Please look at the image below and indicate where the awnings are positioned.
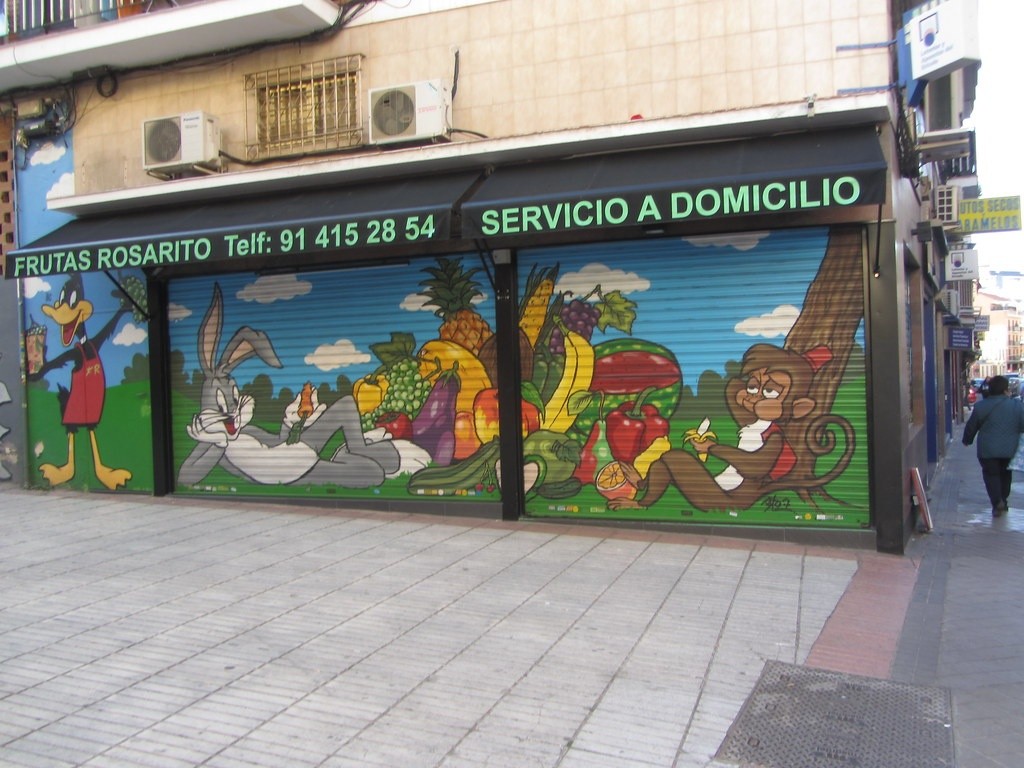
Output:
[4,167,486,281]
[455,122,887,250]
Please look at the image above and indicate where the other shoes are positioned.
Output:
[992,502,1008,517]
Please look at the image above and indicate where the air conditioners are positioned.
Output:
[367,80,454,148]
[934,184,965,226]
[141,109,224,176]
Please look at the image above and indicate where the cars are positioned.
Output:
[967,373,1024,405]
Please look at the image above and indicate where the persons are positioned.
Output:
[962,375,1024,517]
[978,375,991,400]
